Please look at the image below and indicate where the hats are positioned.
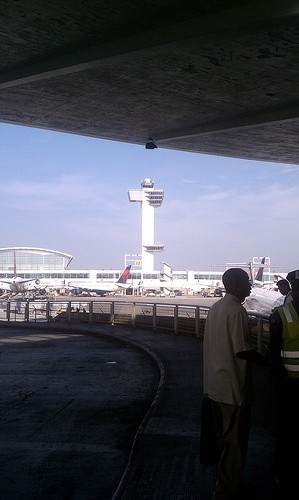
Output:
[286,270,299,285]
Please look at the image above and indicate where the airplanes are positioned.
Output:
[0,275,40,301]
[41,264,135,299]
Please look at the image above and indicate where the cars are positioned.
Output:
[145,291,175,299]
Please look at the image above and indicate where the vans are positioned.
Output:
[213,289,223,298]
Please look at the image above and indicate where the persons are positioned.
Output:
[202,269,270,500]
[268,270,299,500]
[276,279,292,304]
